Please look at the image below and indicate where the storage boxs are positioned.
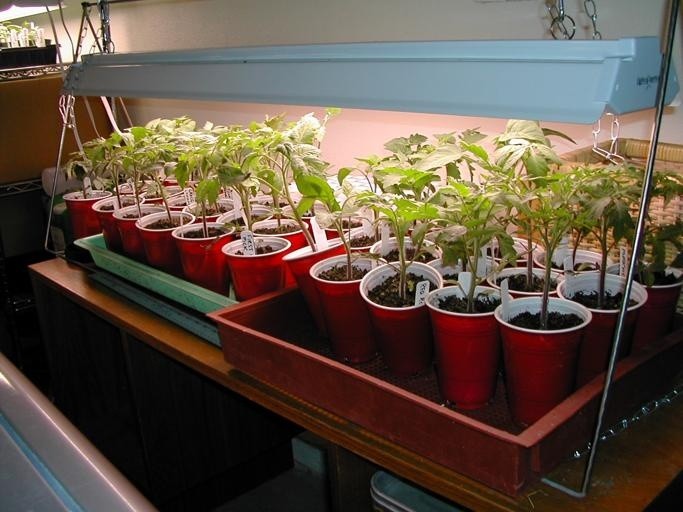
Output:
[0,40,61,69]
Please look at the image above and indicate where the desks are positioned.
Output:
[27,257,681,511]
